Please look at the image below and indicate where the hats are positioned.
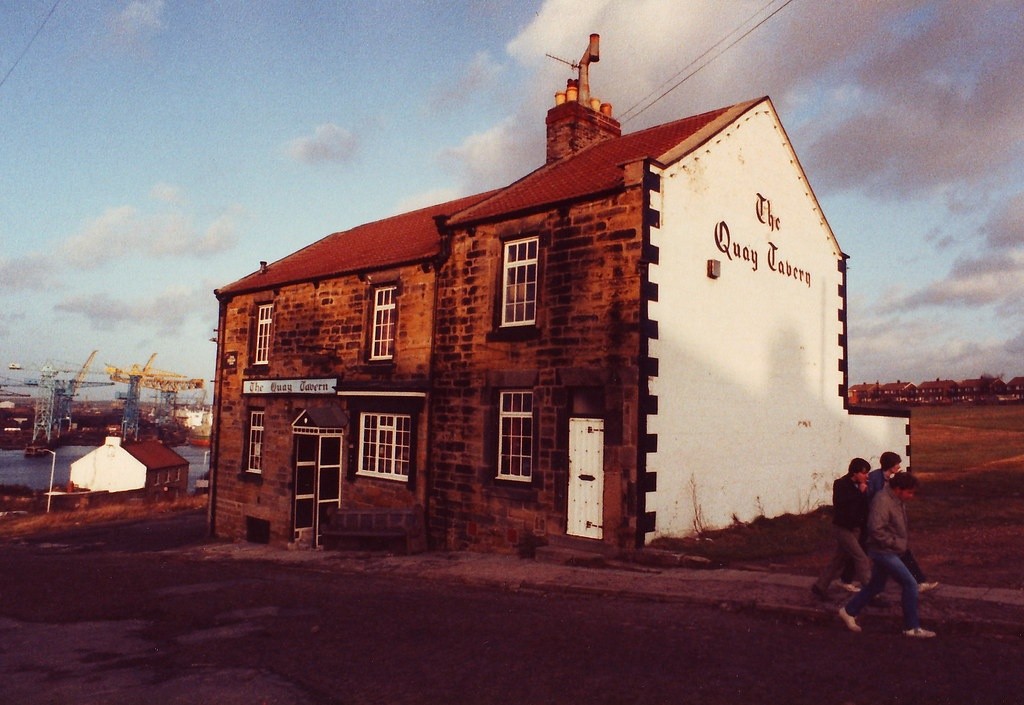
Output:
[881,452,902,470]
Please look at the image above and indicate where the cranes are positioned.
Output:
[1,350,205,459]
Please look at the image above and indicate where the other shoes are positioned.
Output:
[812,584,832,602]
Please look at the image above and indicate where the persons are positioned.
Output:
[839,473,936,637]
[839,452,939,593]
[811,458,892,606]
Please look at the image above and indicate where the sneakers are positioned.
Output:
[902,627,936,639]
[918,581,938,593]
[836,579,861,592]
[837,608,862,632]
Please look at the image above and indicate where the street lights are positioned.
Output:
[41,448,56,515]
